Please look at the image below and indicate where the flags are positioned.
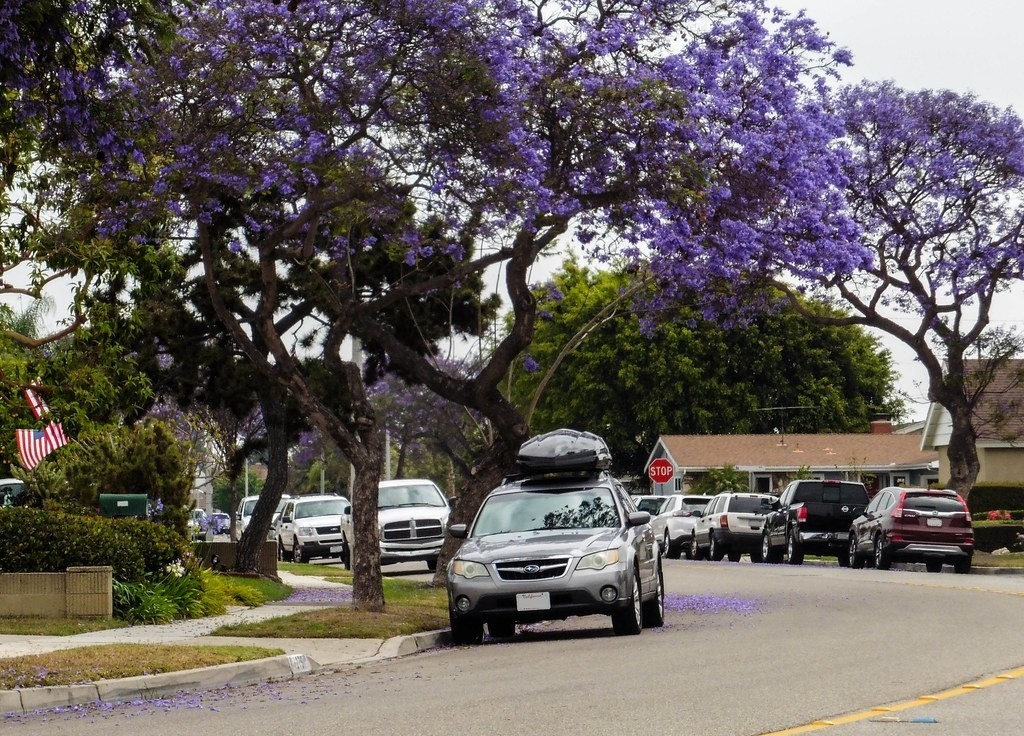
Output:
[15,430,69,473]
[25,379,71,450]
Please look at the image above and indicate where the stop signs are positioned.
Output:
[648,457,673,485]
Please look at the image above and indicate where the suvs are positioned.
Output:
[761,479,872,569]
[235,493,292,544]
[690,490,779,563]
[446,429,665,644]
[338,478,452,572]
[846,486,976,575]
[270,493,352,564]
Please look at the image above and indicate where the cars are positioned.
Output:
[630,493,669,521]
[650,494,714,559]
[212,512,232,533]
[188,509,210,537]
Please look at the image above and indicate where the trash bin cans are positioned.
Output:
[99,493,148,520]
